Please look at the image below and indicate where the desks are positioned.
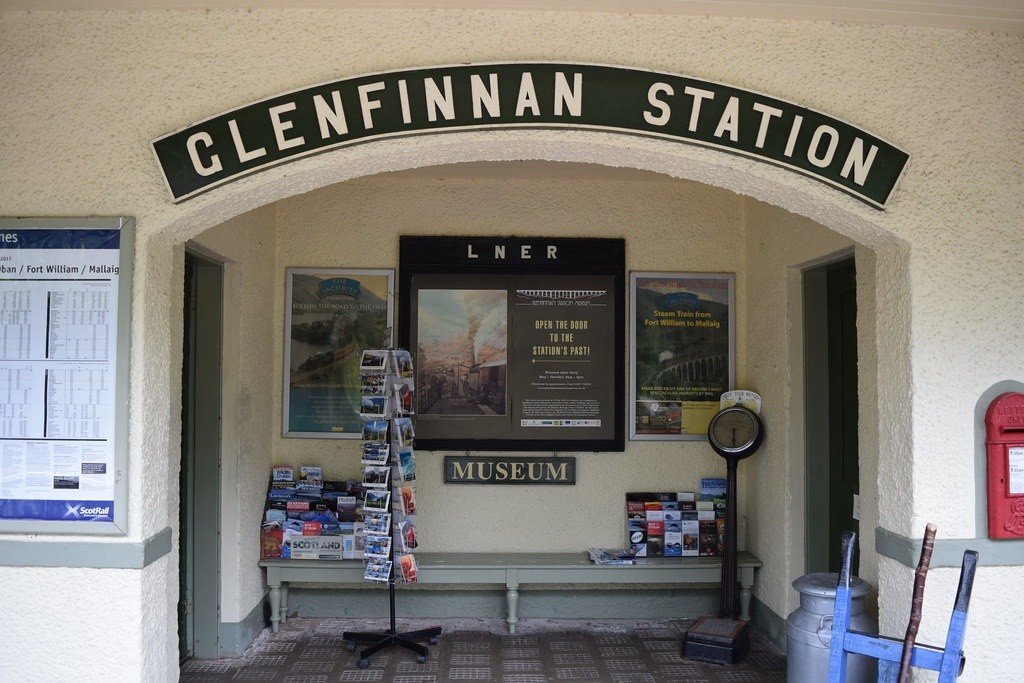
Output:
[259,551,763,634]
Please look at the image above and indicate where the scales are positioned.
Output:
[680,389,766,668]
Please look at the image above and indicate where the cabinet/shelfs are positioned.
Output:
[260,476,369,565]
[623,492,725,558]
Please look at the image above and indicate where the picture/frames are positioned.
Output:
[629,270,736,442]
[281,267,395,440]
[0,215,135,536]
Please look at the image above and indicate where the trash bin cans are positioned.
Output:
[784,572,879,683]
